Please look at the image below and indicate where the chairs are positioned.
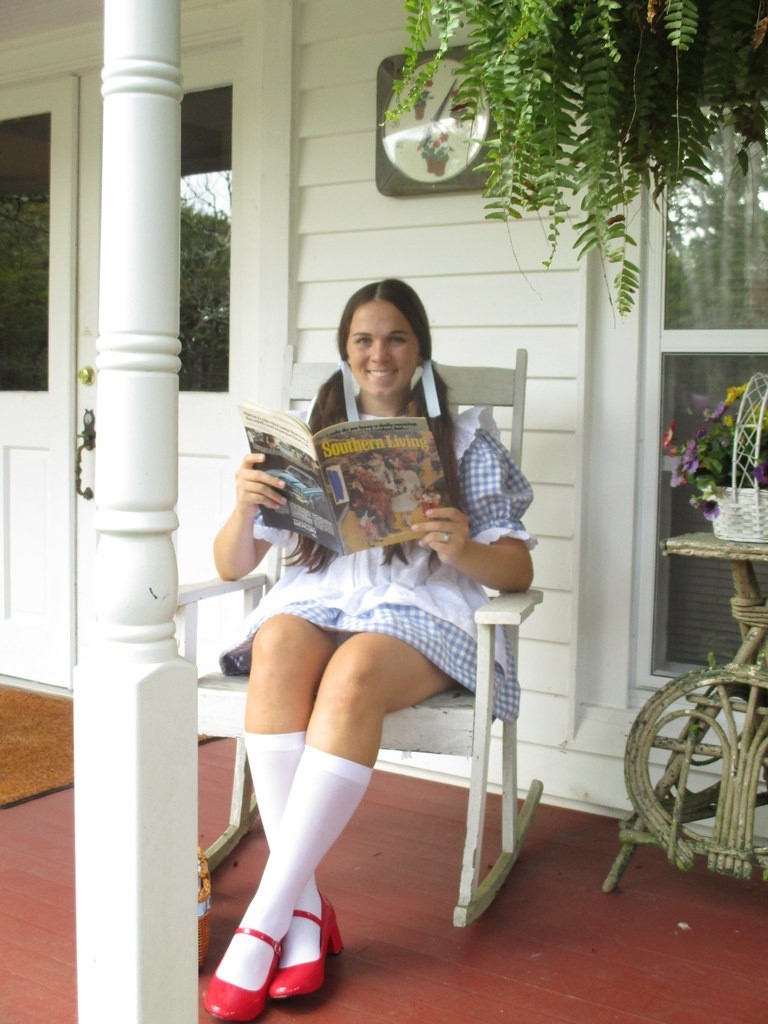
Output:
[176,337,555,943]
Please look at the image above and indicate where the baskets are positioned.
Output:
[198,846,212,969]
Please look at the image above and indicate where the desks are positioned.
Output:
[598,533,768,901]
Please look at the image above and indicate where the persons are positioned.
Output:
[203,279,534,1024]
[349,450,442,547]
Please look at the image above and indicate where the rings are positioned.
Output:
[443,532,450,544]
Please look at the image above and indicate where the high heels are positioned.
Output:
[268,890,344,999]
[204,927,283,1023]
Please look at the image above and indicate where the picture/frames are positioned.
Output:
[362,43,527,201]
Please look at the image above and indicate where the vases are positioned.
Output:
[695,370,768,543]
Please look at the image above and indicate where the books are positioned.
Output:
[236,398,454,557]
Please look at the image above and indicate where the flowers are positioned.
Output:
[663,390,738,517]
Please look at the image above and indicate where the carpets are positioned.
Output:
[174,720,230,749]
[0,680,75,815]
